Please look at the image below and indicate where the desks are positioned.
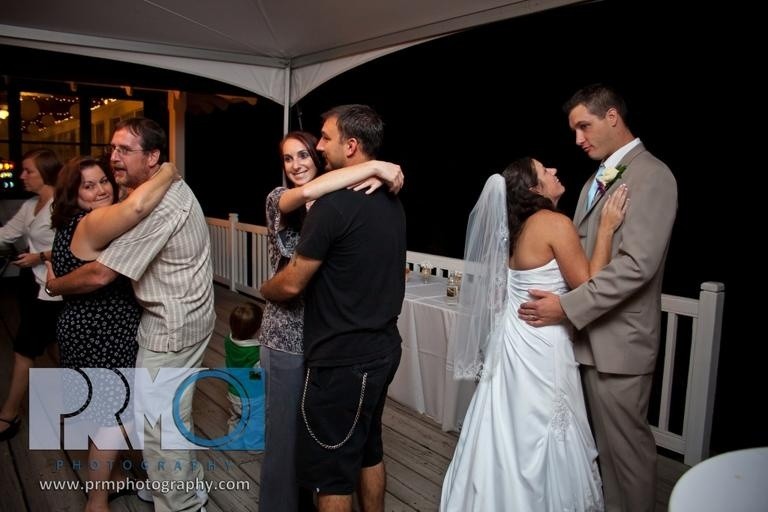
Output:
[383,271,485,435]
[667,446,768,512]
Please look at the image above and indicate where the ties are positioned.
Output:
[585,163,607,207]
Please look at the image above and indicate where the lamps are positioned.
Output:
[0,104,10,121]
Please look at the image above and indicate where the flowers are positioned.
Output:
[591,166,629,197]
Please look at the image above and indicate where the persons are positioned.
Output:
[438,156,630,512]
[518,83,678,512]
[223,301,264,444]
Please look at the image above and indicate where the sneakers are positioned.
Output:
[137,479,209,506]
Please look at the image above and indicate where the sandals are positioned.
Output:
[0,413,22,440]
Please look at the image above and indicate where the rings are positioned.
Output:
[532,315,536,320]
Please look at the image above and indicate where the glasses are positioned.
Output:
[110,145,142,154]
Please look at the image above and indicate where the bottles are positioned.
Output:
[446,269,457,297]
[421,264,433,285]
[406,262,410,283]
[455,273,462,289]
